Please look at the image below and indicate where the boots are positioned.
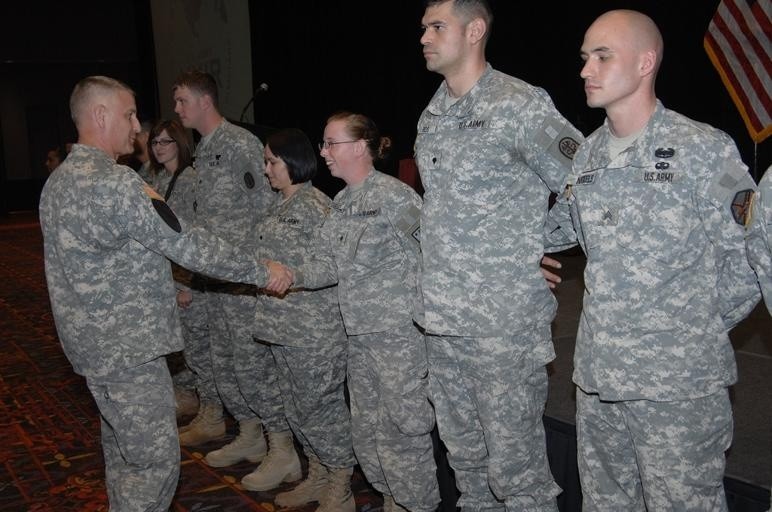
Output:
[177,406,227,448]
[275,456,356,512]
[174,391,199,415]
[241,430,303,492]
[205,416,266,468]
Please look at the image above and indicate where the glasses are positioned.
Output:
[147,140,176,146]
[318,141,361,151]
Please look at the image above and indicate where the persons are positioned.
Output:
[65,140,76,155]
[45,146,64,174]
[128,118,155,185]
[414,2,595,512]
[138,120,200,421]
[169,66,305,494]
[535,6,762,512]
[252,129,358,512]
[37,74,293,512]
[266,107,444,511]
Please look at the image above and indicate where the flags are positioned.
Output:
[703,0,770,146]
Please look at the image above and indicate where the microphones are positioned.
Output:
[240,83,268,122]
